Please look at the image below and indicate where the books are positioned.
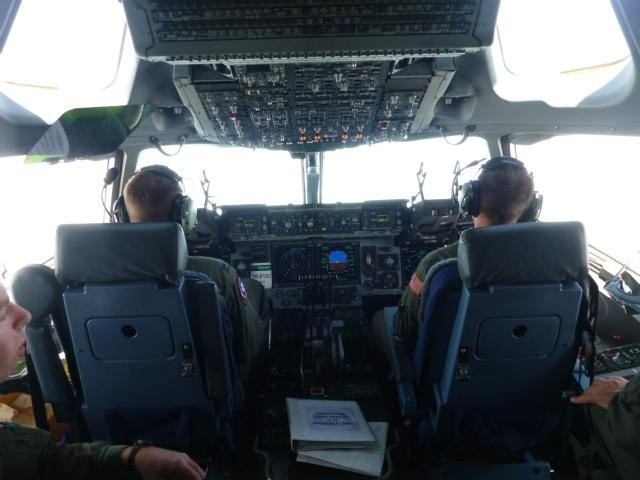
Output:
[285,397,377,453]
[296,421,389,478]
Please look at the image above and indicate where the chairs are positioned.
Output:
[13,222,281,451]
[383,220,594,452]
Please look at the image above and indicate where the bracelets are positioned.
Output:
[124,437,157,480]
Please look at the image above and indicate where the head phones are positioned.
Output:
[459,157,543,223]
[113,166,198,234]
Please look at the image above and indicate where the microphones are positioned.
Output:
[100,168,119,218]
[455,211,472,235]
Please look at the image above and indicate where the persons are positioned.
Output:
[0,279,207,480]
[112,164,286,451]
[358,154,609,415]
[531,370,640,479]
